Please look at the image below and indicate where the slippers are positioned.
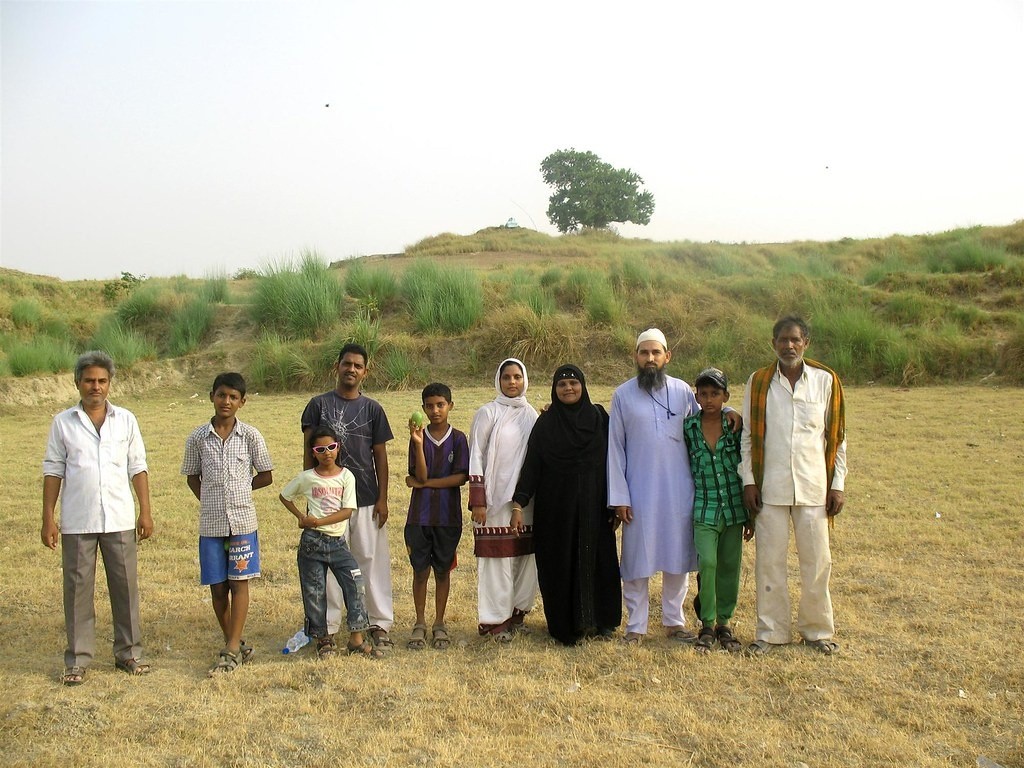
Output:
[622,635,644,646]
[665,626,697,643]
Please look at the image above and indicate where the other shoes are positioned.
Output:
[598,627,612,637]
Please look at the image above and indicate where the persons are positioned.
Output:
[510,364,622,647]
[467,358,540,643]
[404,384,469,650]
[41,349,154,686]
[180,372,273,677]
[683,367,754,651]
[607,327,743,648]
[301,345,395,655]
[737,316,848,657]
[279,426,385,660]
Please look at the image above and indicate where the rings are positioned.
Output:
[616,515,619,518]
[513,527,517,531]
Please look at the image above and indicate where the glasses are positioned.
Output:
[313,443,338,454]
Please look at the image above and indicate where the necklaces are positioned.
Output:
[645,382,677,420]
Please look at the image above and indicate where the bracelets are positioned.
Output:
[313,518,317,528]
[512,508,522,513]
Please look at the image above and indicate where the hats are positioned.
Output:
[695,368,727,391]
[635,328,667,351]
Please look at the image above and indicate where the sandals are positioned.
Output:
[514,623,530,634]
[806,639,840,653]
[407,624,428,650]
[431,625,451,649]
[715,625,740,651]
[116,658,150,673]
[347,639,386,658]
[492,629,513,642]
[207,650,243,677]
[63,668,86,682]
[316,639,337,656]
[749,640,770,656]
[369,628,395,648]
[240,638,255,661]
[694,628,715,654]
